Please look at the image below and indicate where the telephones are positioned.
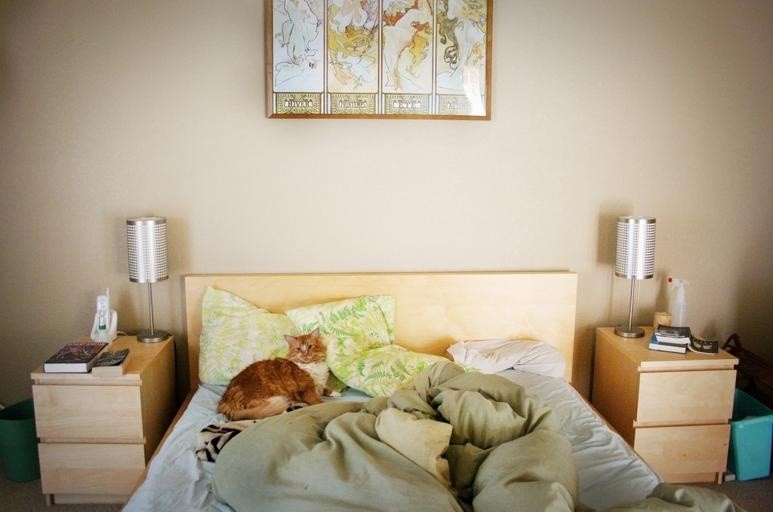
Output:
[90,288,118,342]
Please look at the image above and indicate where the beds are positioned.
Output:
[111,272,749,512]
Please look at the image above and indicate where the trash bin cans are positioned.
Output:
[0,399,40,483]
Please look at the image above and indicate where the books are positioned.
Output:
[91,347,131,376]
[41,342,111,373]
[647,324,720,355]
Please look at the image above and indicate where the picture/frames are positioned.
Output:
[263,0,493,122]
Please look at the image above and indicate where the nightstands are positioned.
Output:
[28,330,176,506]
[590,324,740,485]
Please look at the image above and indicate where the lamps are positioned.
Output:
[125,217,170,344]
[612,215,655,338]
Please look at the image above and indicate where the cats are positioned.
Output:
[217,327,343,421]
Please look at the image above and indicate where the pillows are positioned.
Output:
[446,338,567,379]
[286,295,476,399]
[198,283,395,392]
